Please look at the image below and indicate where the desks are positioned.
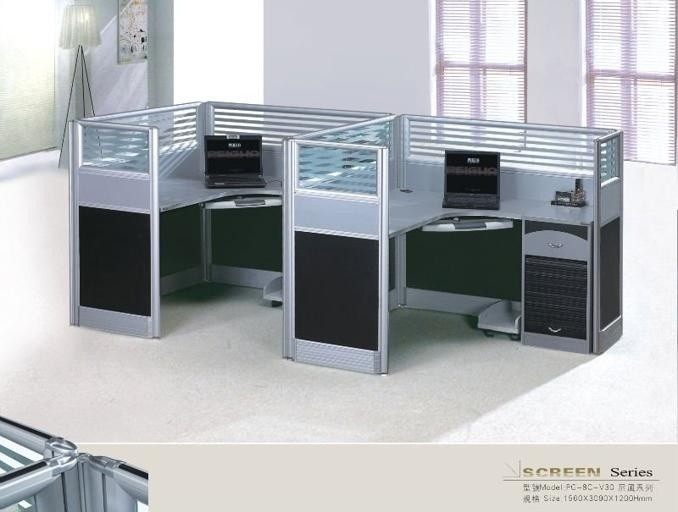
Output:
[157,176,597,379]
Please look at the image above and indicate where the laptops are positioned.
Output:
[442,149,501,210]
[203,133,266,188]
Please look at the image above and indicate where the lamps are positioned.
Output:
[58,3,102,170]
